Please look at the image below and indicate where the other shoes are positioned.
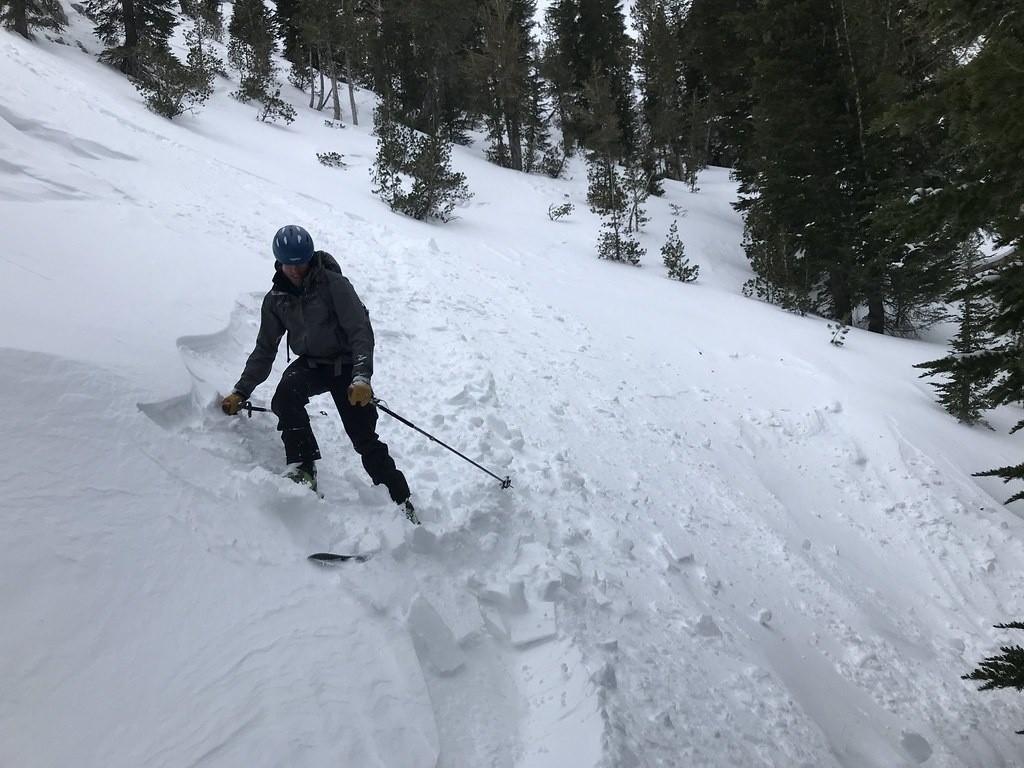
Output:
[281,460,317,492]
[398,501,419,525]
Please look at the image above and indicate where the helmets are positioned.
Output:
[272,225,314,265]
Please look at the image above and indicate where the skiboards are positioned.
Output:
[306,489,370,563]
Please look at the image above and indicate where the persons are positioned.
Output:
[220,225,424,527]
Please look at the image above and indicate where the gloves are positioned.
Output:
[348,381,372,406]
[221,393,246,415]
